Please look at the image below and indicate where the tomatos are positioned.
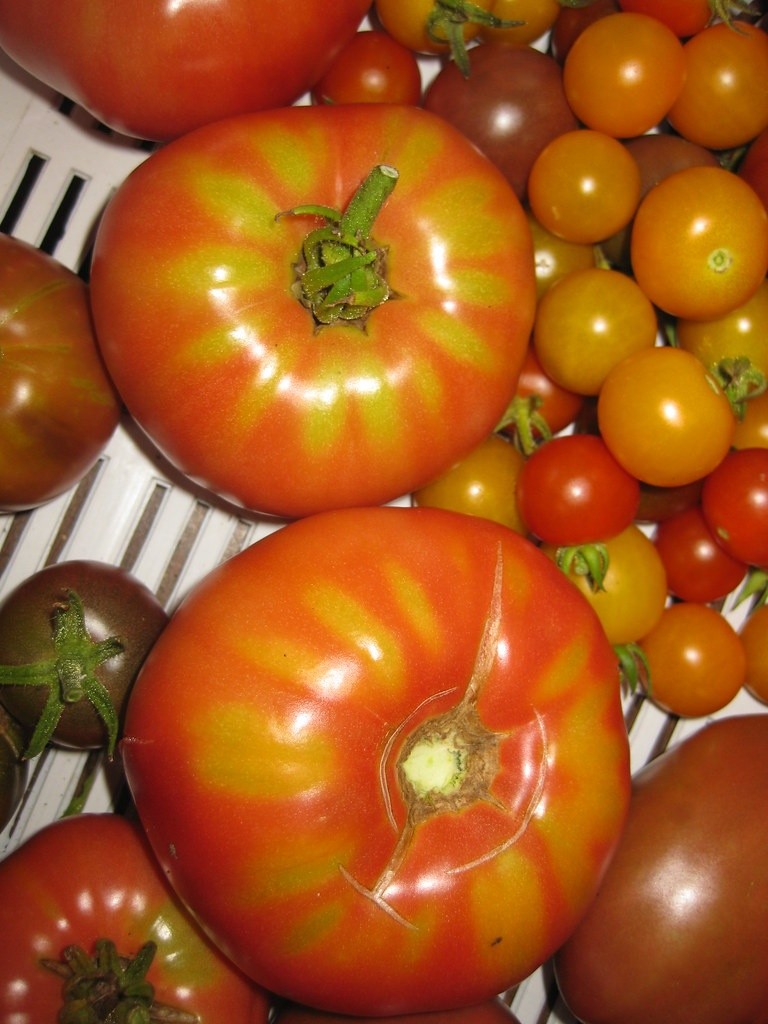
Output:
[0,0,768,1024]
